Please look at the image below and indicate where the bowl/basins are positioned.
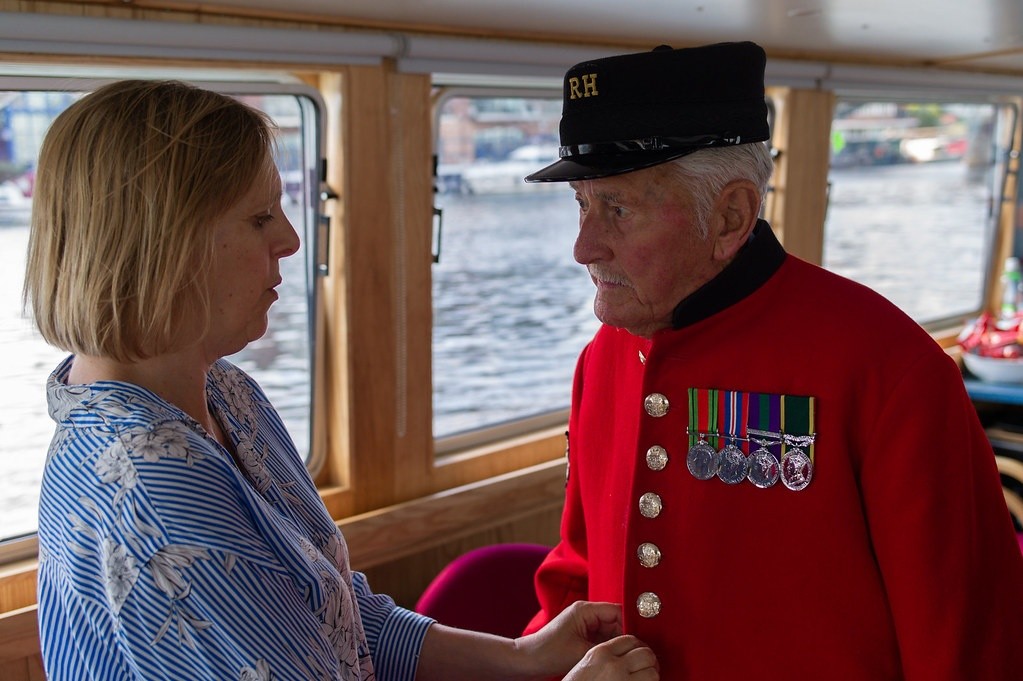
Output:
[960,348,1023,383]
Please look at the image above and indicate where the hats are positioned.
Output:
[523,40,770,182]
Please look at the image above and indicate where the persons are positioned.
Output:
[22,80,661,681]
[524,42,1023,681]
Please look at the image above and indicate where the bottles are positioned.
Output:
[994,257,1023,330]
